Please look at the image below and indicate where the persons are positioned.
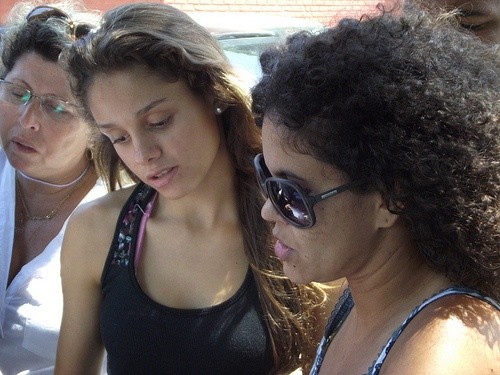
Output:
[1,2,136,374]
[252,11,499,373]
[53,1,349,375]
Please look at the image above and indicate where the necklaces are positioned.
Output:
[14,160,92,236]
[405,0,500,49]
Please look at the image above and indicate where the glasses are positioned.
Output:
[254,152,370,230]
[0,78,87,123]
[26,5,98,42]
